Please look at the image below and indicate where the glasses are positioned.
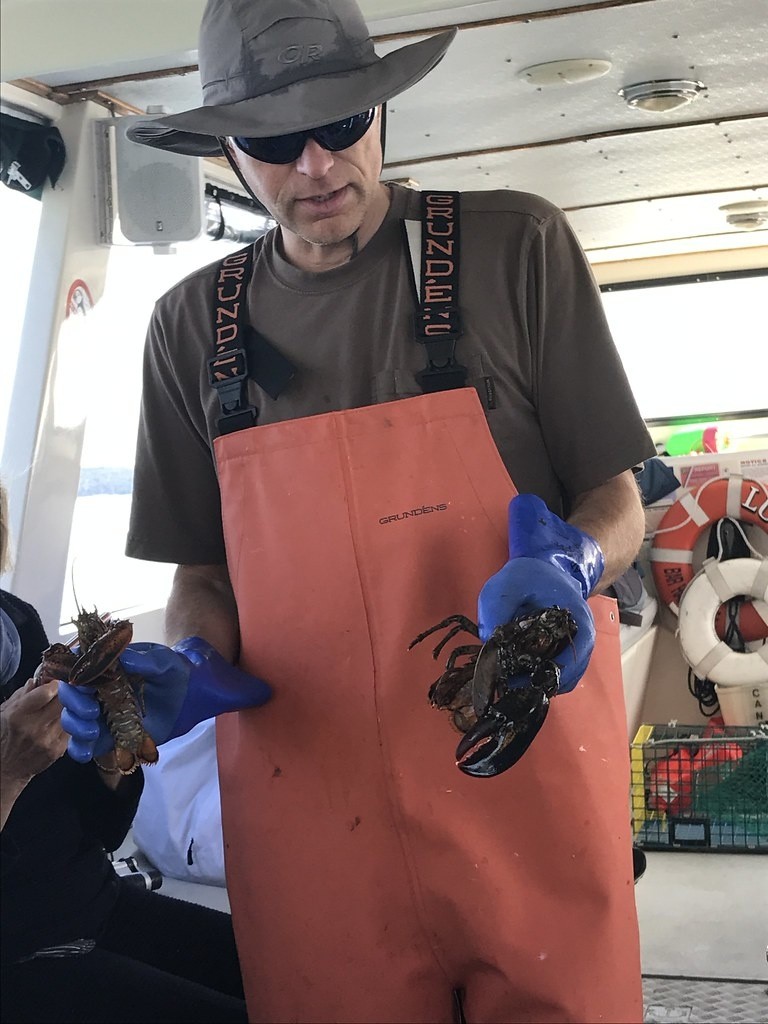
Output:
[233,105,376,164]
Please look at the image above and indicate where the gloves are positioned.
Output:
[57,636,273,762]
[477,493,605,696]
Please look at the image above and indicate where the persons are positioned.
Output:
[125,0,657,1024]
[0,481,250,1024]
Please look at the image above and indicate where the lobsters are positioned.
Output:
[33,576,161,778]
[406,604,578,779]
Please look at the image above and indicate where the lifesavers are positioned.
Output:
[651,476,768,642]
[677,557,768,685]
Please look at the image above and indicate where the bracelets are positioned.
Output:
[95,760,117,772]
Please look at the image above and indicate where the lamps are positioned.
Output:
[722,202,768,232]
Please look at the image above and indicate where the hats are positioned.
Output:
[128,0,457,156]
